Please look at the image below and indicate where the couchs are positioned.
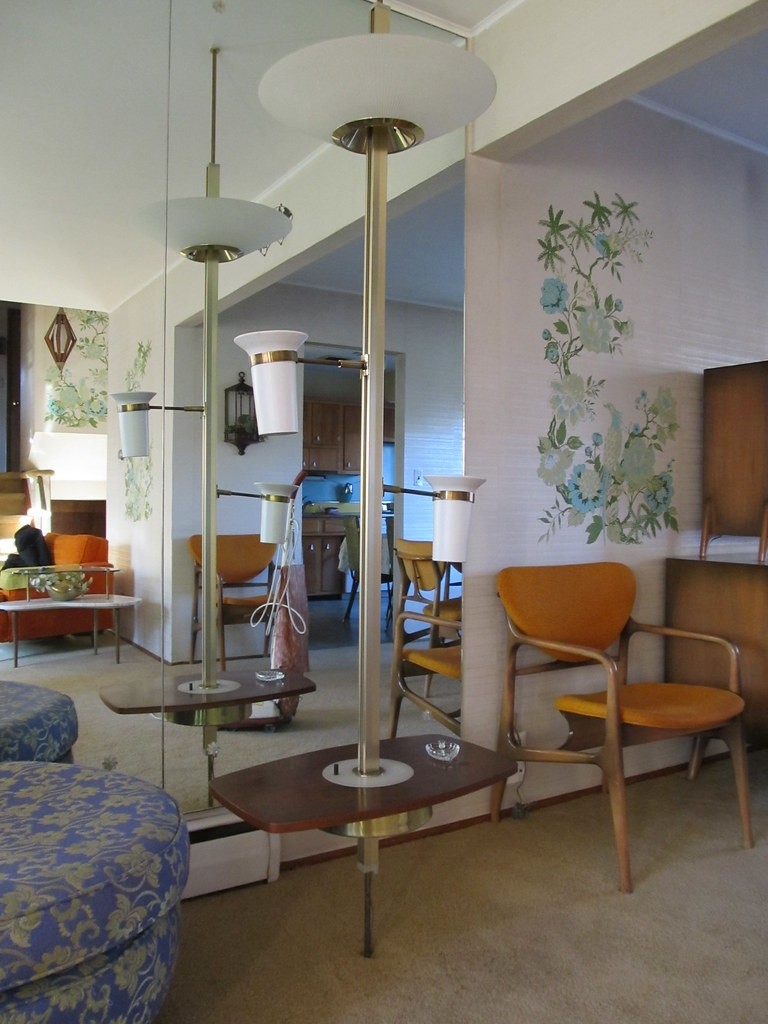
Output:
[0,762,190,1023]
[0,677,78,764]
[0,533,115,650]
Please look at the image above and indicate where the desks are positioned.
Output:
[1,566,143,669]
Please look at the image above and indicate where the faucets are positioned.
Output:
[302,495,314,513]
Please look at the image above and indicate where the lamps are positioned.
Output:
[387,473,485,564]
[141,197,293,264]
[217,482,299,545]
[253,33,500,160]
[108,392,206,460]
[234,328,363,437]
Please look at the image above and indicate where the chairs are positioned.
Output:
[493,560,756,894]
[385,538,462,737]
[189,534,284,671]
